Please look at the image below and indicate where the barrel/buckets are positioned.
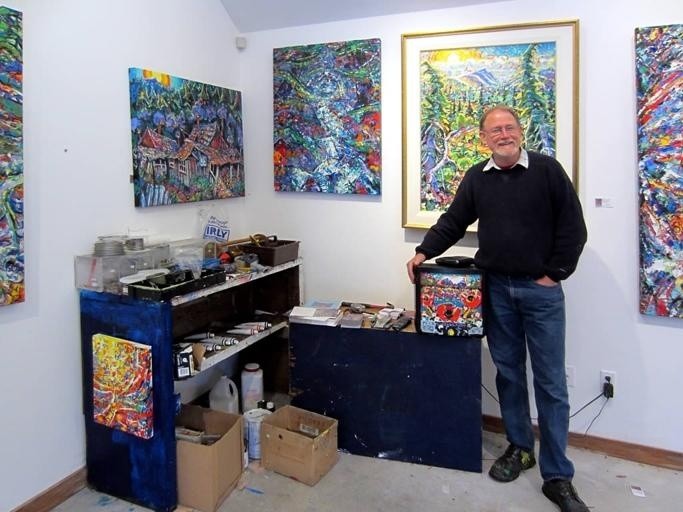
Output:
[209,373,238,417]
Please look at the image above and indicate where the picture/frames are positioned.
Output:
[633,22,683,318]
[128,68,247,209]
[270,35,385,196]
[0,1,28,304]
[399,19,582,236]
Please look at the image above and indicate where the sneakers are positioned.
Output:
[541,477,590,511]
[489,444,536,482]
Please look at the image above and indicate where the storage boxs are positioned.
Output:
[259,402,341,489]
[174,405,246,512]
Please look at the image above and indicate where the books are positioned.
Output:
[289,305,343,327]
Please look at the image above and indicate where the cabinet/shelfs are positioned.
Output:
[76,254,305,512]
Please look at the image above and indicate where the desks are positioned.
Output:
[286,308,484,474]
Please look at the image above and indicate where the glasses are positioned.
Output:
[486,124,522,135]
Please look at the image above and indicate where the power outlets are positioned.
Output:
[565,363,575,389]
[599,371,615,393]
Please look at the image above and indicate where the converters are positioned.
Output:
[603,383,613,397]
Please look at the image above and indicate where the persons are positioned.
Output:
[408,106,589,512]
